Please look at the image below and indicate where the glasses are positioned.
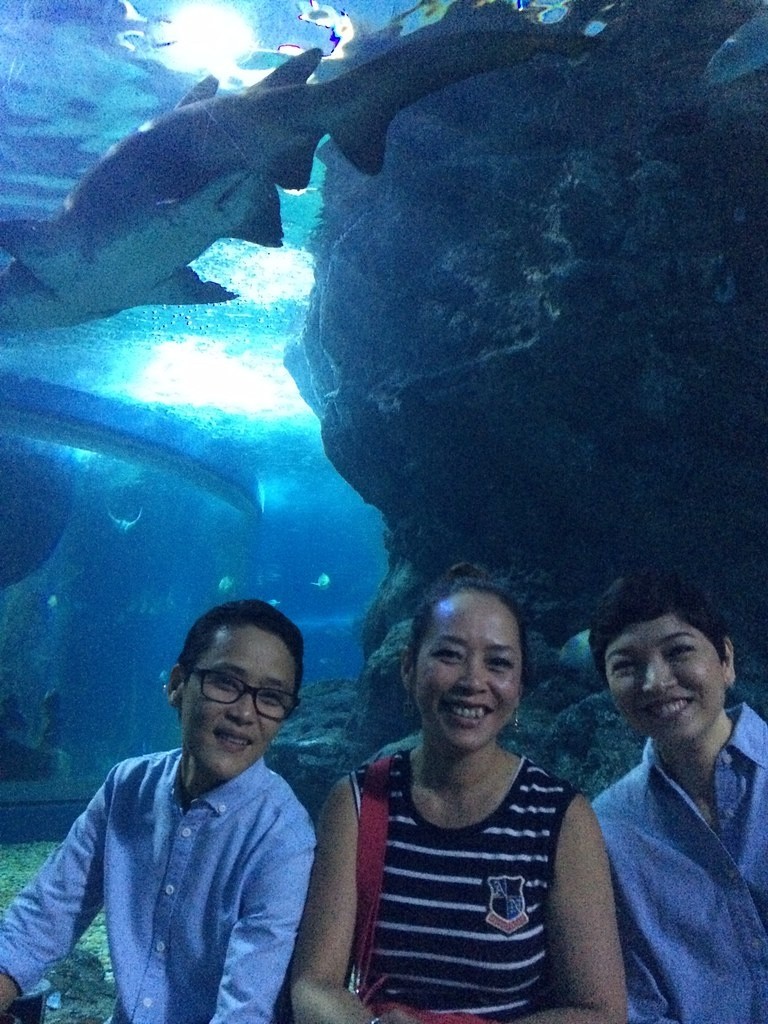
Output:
[182,665,301,721]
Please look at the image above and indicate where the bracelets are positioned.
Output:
[370,1018,380,1024]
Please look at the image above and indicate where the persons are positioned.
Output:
[286,580,628,1024]
[588,572,768,1024]
[3,599,316,1024]
